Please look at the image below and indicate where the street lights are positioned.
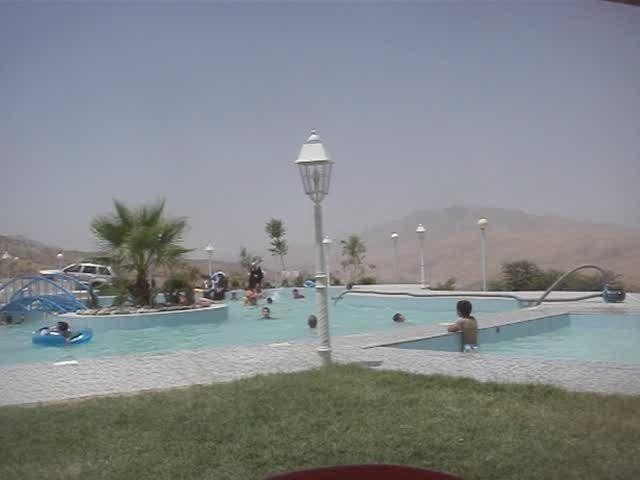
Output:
[416,224,426,288]
[477,218,488,291]
[391,232,399,284]
[295,130,334,359]
[204,243,214,276]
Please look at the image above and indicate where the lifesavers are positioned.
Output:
[31,327,92,345]
[303,280,314,287]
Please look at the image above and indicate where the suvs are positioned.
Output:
[39,263,117,285]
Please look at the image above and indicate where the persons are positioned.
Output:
[308,315,317,328]
[230,265,275,307]
[448,299,480,353]
[143,284,157,307]
[393,313,405,322]
[51,321,83,343]
[257,308,273,319]
[292,289,304,299]
